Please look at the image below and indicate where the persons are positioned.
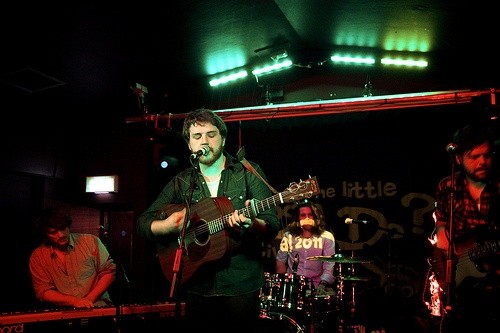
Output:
[29,206,116,309]
[432,125,500,333]
[276,202,336,295]
[137,108,282,333]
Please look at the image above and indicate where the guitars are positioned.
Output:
[431,225,500,294]
[155,173,321,285]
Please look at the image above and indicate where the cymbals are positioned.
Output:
[306,253,374,263]
[343,275,368,281]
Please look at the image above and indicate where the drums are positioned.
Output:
[259,271,340,333]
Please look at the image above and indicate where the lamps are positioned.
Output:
[85,175,118,240]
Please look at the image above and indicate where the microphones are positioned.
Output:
[344,218,370,224]
[445,143,459,153]
[190,146,209,159]
[293,254,298,270]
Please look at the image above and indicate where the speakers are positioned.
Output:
[107,211,138,277]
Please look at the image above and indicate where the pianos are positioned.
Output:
[0,299,186,333]
